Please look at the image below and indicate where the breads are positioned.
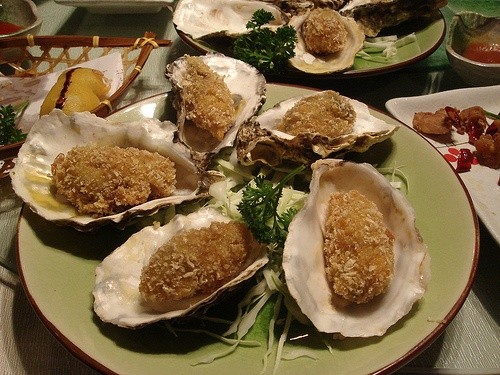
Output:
[39,67,111,116]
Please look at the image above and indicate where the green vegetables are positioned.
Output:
[237,164,306,247]
[234,8,297,75]
[0,100,27,146]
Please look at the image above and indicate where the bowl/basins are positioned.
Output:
[0,0,43,61]
[444,10,499,85]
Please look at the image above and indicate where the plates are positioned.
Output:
[15,82,481,374]
[174,0,446,83]
[382,85,500,249]
